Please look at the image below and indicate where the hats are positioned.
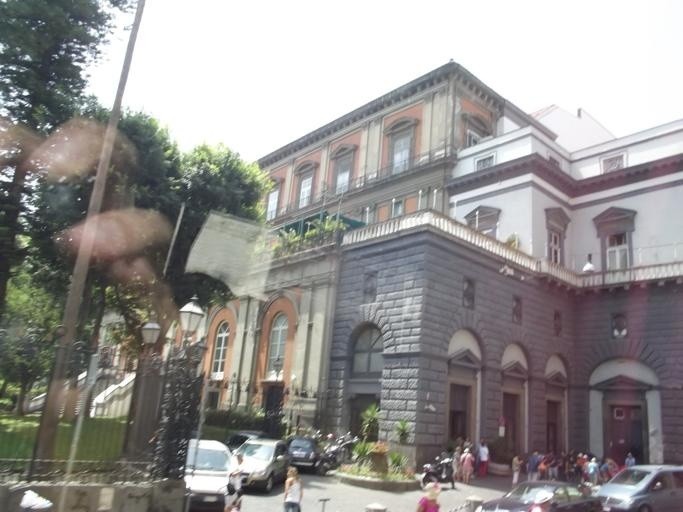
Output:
[577,452,596,462]
[464,448,470,453]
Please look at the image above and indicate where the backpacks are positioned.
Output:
[227,483,236,495]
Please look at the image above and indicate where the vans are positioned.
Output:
[596,464,683,512]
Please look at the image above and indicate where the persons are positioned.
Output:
[511,449,636,488]
[413,482,441,512]
[448,433,493,484]
[284,467,305,512]
[224,451,244,512]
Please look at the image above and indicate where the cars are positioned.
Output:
[184,429,322,512]
[475,464,602,512]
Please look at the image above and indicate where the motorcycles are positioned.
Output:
[422,456,454,489]
